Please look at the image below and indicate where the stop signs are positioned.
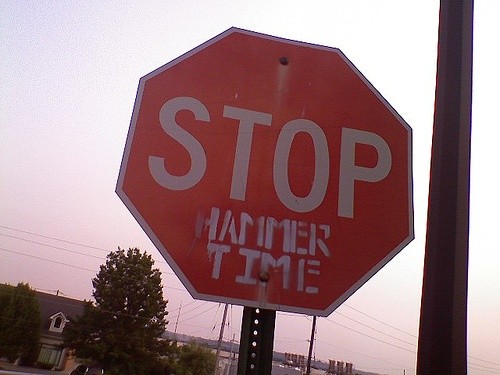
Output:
[114,27,415,316]
